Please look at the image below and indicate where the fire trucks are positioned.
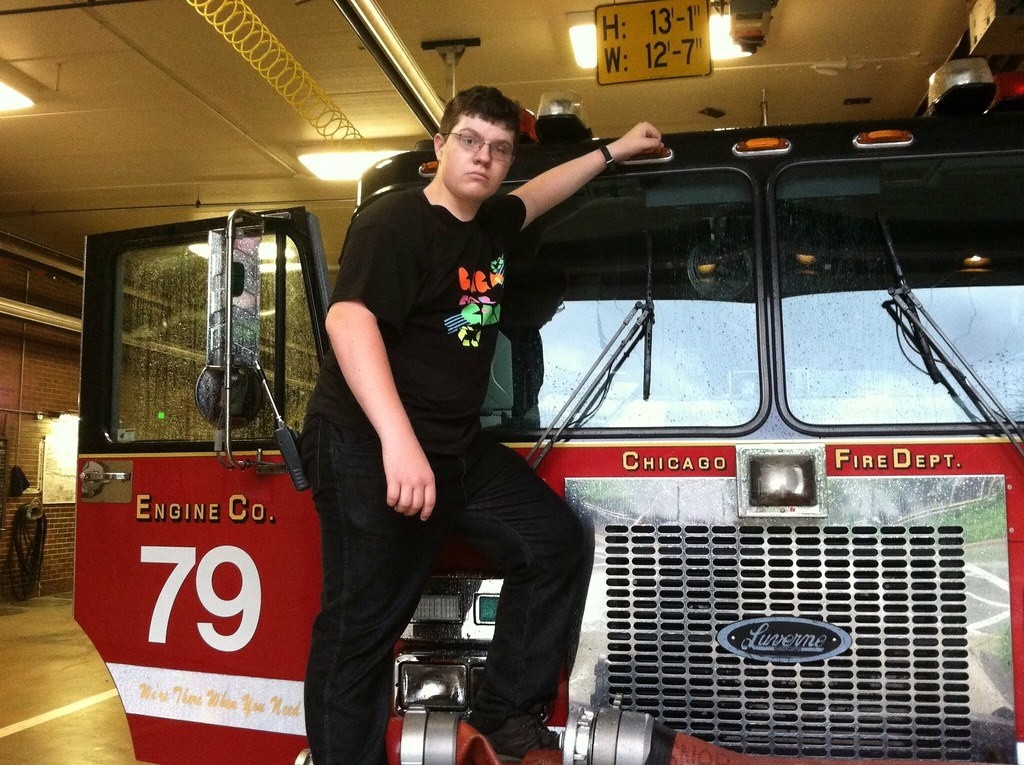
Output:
[73,54,1023,765]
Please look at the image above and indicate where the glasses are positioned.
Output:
[442,132,516,164]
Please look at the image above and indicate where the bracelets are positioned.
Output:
[600,145,614,169]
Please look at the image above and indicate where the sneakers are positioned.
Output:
[467,709,562,760]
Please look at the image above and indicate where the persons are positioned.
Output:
[299,86,663,765]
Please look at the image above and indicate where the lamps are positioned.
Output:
[708,2,752,61]
[567,10,598,68]
